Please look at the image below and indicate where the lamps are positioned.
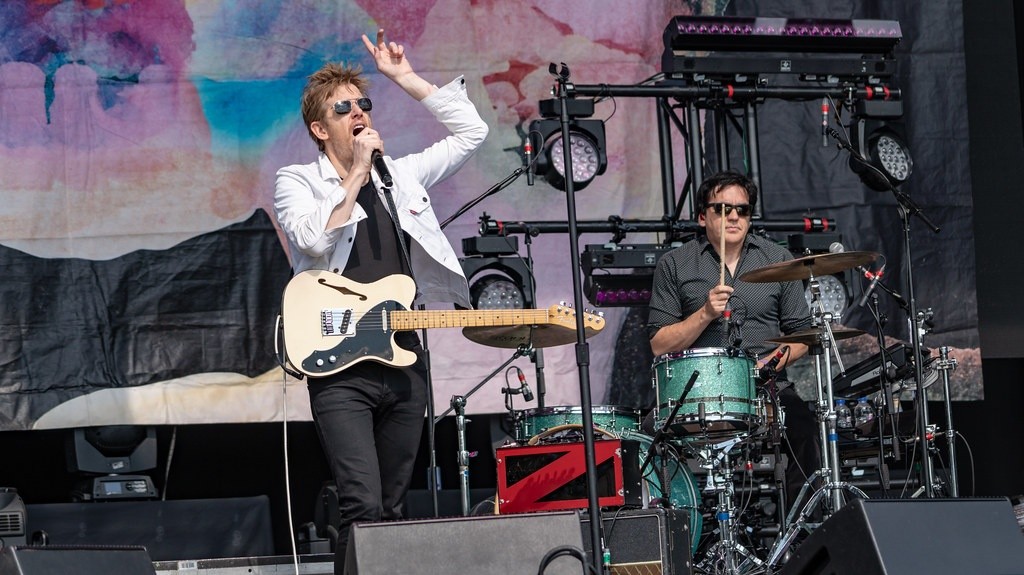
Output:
[526,94,609,190]
[801,270,853,328]
[450,235,531,311]
[847,101,915,189]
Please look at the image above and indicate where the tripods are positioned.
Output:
[693,268,946,575]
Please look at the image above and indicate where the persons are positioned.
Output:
[275,29,490,575]
[646,168,831,522]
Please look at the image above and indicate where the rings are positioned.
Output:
[368,133,370,135]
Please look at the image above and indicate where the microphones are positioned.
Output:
[517,368,533,401]
[759,346,789,380]
[371,149,393,187]
[821,97,829,146]
[524,137,534,186]
[722,300,732,339]
[829,242,877,281]
[859,265,886,308]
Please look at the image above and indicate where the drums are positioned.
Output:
[605,429,702,562]
[508,407,643,438]
[651,348,765,441]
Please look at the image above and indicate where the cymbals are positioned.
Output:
[764,326,868,345]
[460,324,604,349]
[736,247,882,284]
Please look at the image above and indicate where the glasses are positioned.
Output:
[319,98,373,114]
[703,204,753,217]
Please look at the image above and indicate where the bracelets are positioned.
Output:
[759,359,768,364]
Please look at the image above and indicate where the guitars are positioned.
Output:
[280,268,605,379]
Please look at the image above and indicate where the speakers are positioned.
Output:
[581,504,693,575]
[774,495,1024,575]
[344,508,587,575]
[0,544,157,575]
